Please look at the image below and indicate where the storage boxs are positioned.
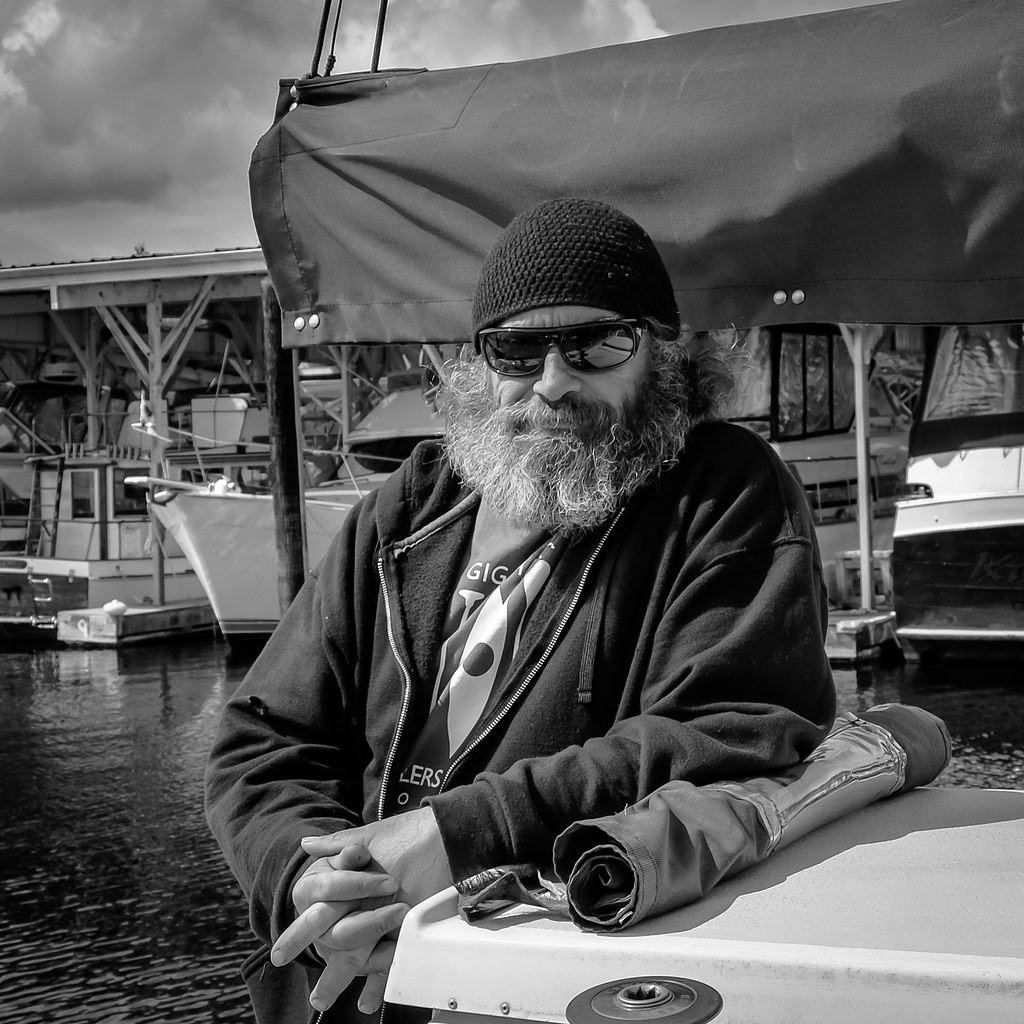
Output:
[834,549,895,611]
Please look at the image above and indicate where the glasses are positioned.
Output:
[478,319,647,376]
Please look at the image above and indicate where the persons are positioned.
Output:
[202,198,838,1024]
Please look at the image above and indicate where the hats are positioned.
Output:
[472,198,681,359]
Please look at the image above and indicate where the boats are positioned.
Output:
[724,322,1024,668]
[122,368,448,663]
[1,338,338,649]
